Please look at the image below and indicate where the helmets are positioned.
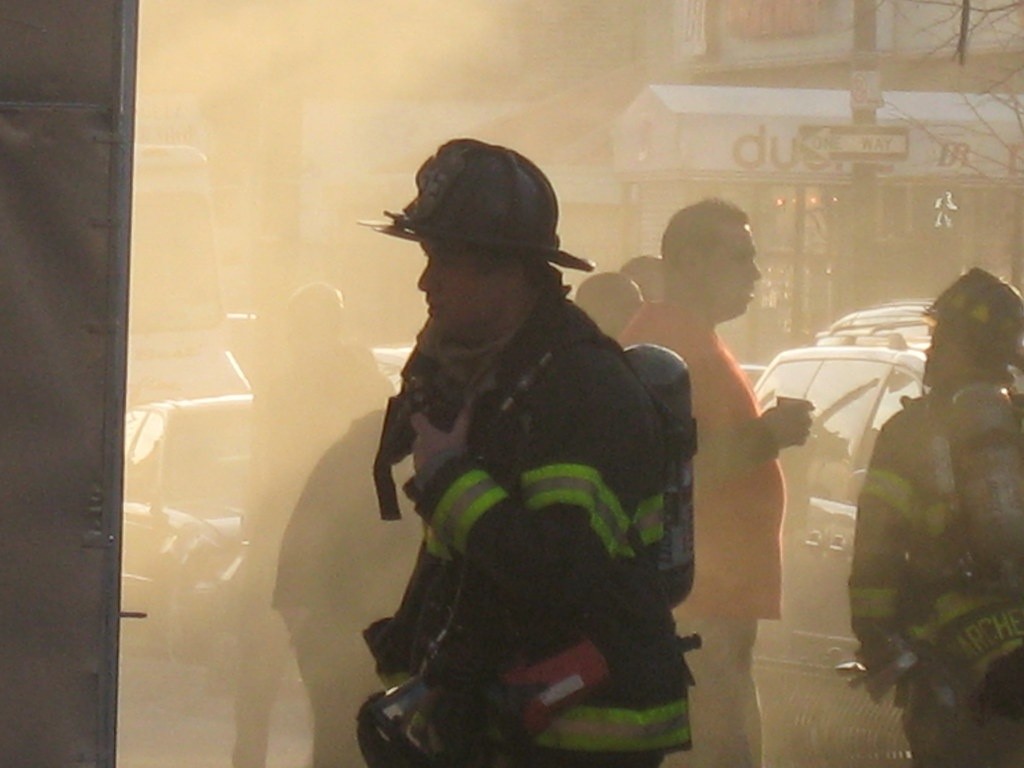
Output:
[356,138,596,273]
[921,267,1024,366]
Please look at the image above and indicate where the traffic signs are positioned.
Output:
[800,122,912,162]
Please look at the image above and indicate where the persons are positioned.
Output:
[618,196,812,768]
[576,255,662,340]
[846,266,1024,768]
[229,283,395,768]
[272,407,425,768]
[355,137,696,768]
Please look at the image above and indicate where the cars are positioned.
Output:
[801,300,1003,363]
[750,347,967,632]
[116,396,307,666]
[737,360,775,393]
[367,345,428,396]
